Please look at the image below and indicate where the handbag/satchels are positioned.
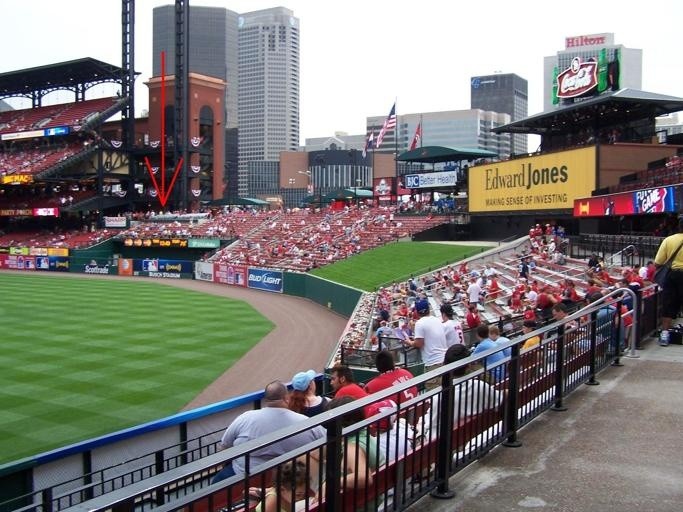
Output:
[653,263,673,290]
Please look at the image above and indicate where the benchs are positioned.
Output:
[463,251,633,323]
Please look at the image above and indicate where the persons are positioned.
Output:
[46,196,76,204]
[64,118,83,125]
[6,114,34,130]
[654,218,683,346]
[0,144,75,175]
[0,205,206,248]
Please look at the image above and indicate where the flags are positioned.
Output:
[361,102,421,160]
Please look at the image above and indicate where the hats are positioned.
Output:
[415,299,428,312]
[292,370,317,392]
[440,304,457,316]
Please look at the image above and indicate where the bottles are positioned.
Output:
[610,47,620,92]
[597,47,609,92]
[552,66,561,106]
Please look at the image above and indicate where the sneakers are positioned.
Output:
[658,331,670,346]
